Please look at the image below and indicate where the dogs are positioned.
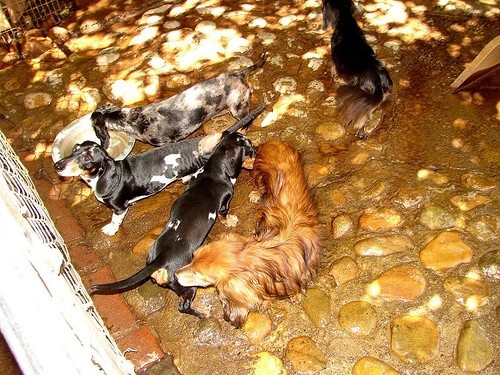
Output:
[174,139,327,330]
[87,130,259,321]
[53,133,237,237]
[90,52,267,151]
[322,0,394,130]
